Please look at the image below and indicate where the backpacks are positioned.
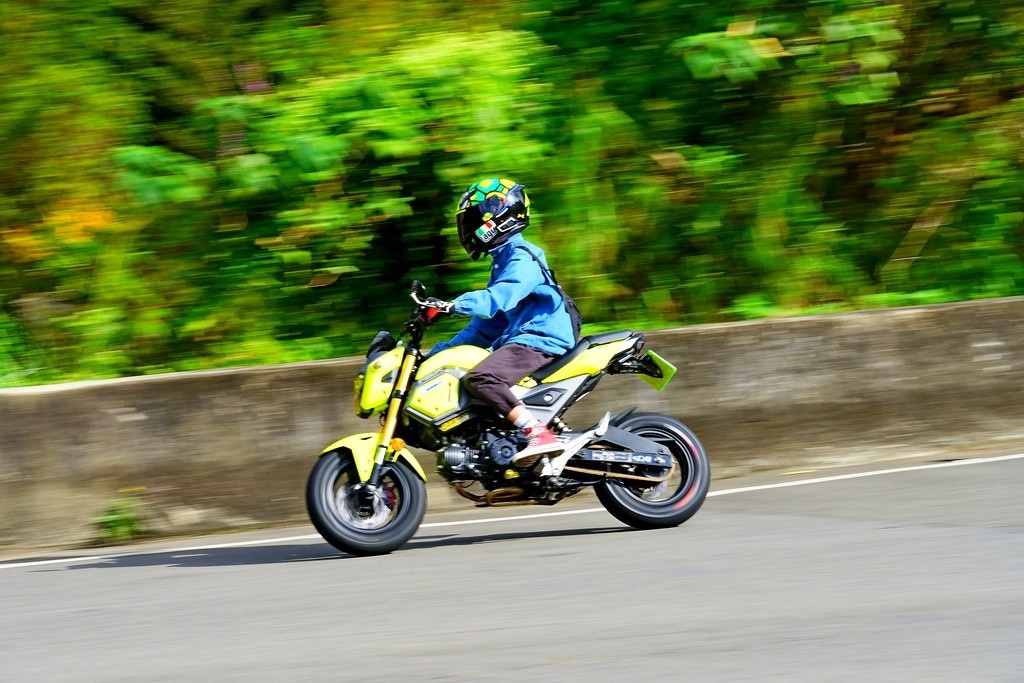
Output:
[504,247,582,350]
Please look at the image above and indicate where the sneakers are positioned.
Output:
[510,424,566,467]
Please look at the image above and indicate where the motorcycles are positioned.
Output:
[302,279,712,556]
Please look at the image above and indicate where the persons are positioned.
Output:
[421,178,582,508]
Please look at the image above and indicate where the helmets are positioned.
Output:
[457,178,529,261]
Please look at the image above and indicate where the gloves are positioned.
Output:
[424,297,454,323]
